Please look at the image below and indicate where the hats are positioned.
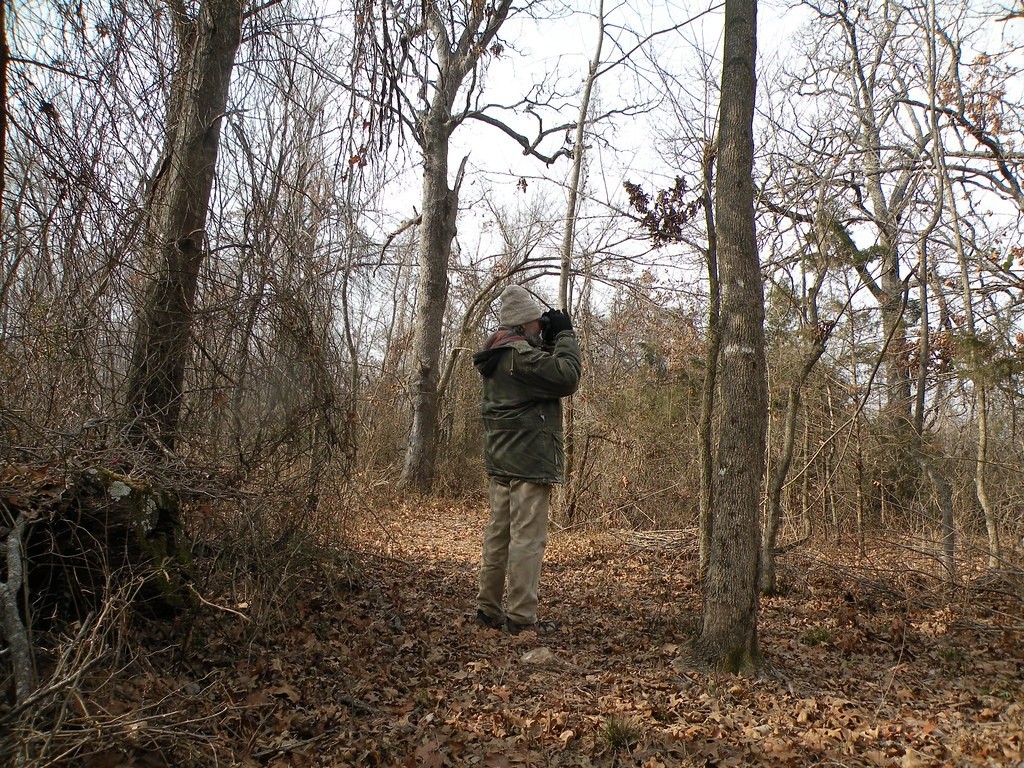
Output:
[498,284,542,330]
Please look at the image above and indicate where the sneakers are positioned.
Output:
[476,609,557,637]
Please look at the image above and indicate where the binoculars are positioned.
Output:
[538,315,552,330]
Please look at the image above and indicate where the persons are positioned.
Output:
[477,284,582,635]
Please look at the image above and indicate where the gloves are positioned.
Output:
[541,308,573,343]
